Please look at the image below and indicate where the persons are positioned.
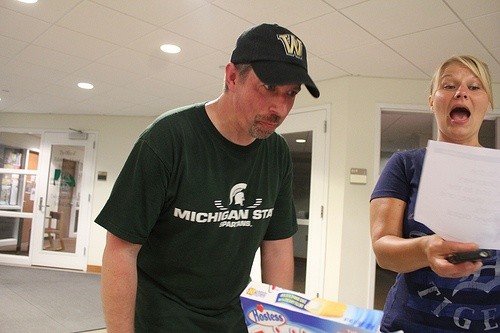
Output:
[369,54,500,333]
[94,24,320,332]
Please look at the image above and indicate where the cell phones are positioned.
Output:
[447,250,491,263]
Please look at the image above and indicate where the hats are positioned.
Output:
[230,23,320,99]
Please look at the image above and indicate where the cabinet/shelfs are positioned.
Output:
[0,144,30,251]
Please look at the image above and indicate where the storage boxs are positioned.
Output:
[239,281,384,333]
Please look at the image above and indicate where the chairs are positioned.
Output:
[45,212,65,251]
